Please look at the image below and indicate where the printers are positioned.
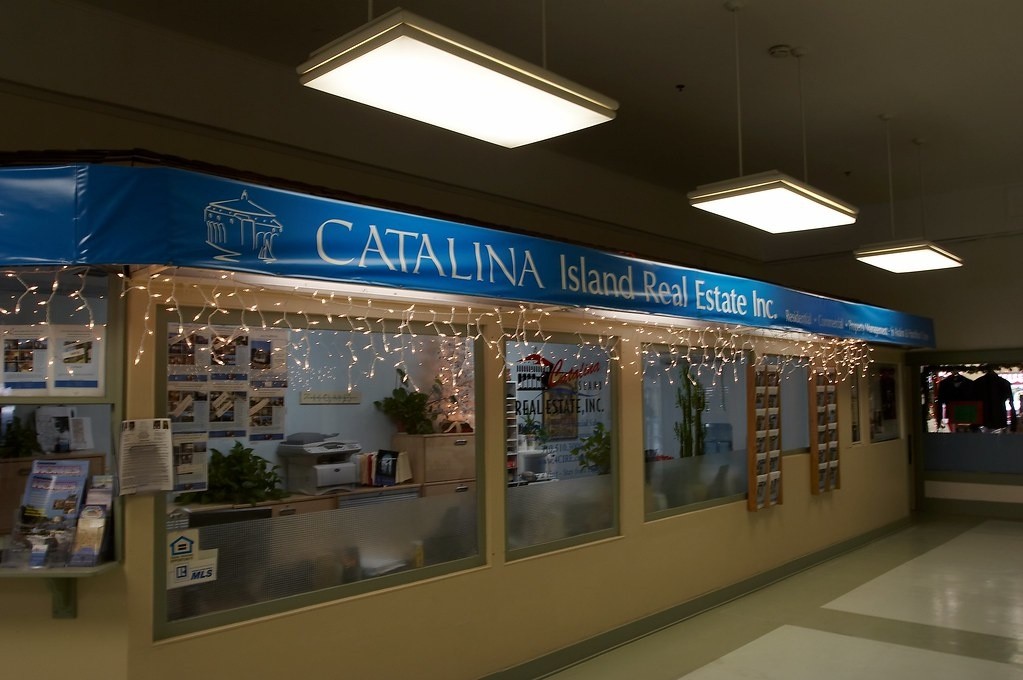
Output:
[277,432,362,497]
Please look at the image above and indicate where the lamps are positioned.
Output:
[687,1,861,235]
[296,0,621,149]
[854,112,963,274]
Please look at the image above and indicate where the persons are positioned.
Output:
[935,368,1016,433]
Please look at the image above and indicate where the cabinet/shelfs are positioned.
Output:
[166,432,477,622]
[0,451,107,537]
[506,381,519,487]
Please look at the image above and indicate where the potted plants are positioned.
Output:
[207,444,280,504]
[518,416,548,451]
[373,369,436,435]
